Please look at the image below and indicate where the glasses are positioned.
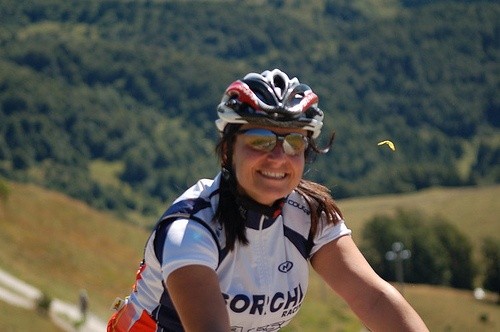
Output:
[232,128,310,157]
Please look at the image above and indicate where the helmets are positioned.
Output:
[216,68,323,139]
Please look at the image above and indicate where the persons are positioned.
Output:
[107,68,428,332]
